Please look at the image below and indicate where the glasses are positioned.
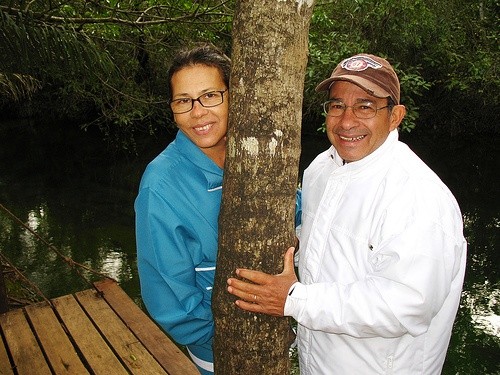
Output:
[323,98,390,119]
[168,88,228,114]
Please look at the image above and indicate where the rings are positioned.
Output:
[254,295,257,302]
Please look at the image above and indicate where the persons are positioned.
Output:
[226,53,467,375]
[135,43,303,375]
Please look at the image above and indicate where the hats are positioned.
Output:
[315,53,400,105]
